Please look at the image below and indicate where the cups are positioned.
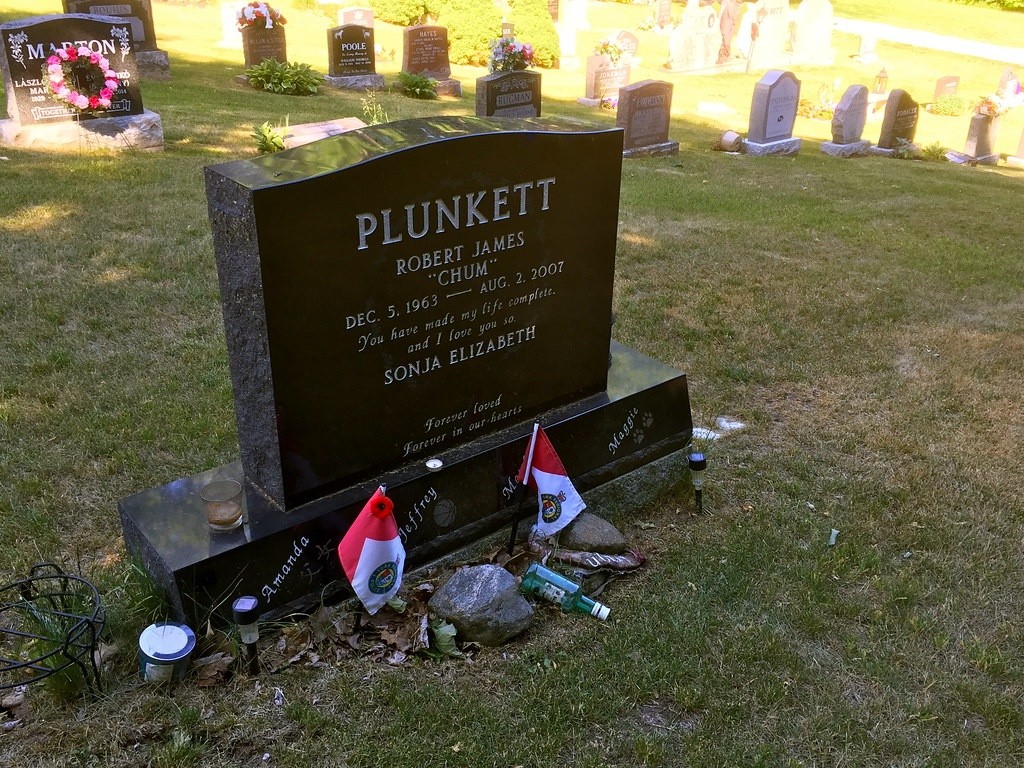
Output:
[199,480,243,531]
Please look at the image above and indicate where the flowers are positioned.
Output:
[592,39,622,66]
[971,92,1007,119]
[235,1,288,30]
[41,45,121,114]
[602,97,620,110]
[1005,80,1024,94]
[487,36,537,74]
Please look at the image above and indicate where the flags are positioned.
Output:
[517,428,587,538]
[339,488,406,613]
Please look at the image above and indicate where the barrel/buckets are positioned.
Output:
[719,129,742,152]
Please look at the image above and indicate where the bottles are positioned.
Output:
[520,560,611,622]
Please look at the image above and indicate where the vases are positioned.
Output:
[513,62,529,71]
